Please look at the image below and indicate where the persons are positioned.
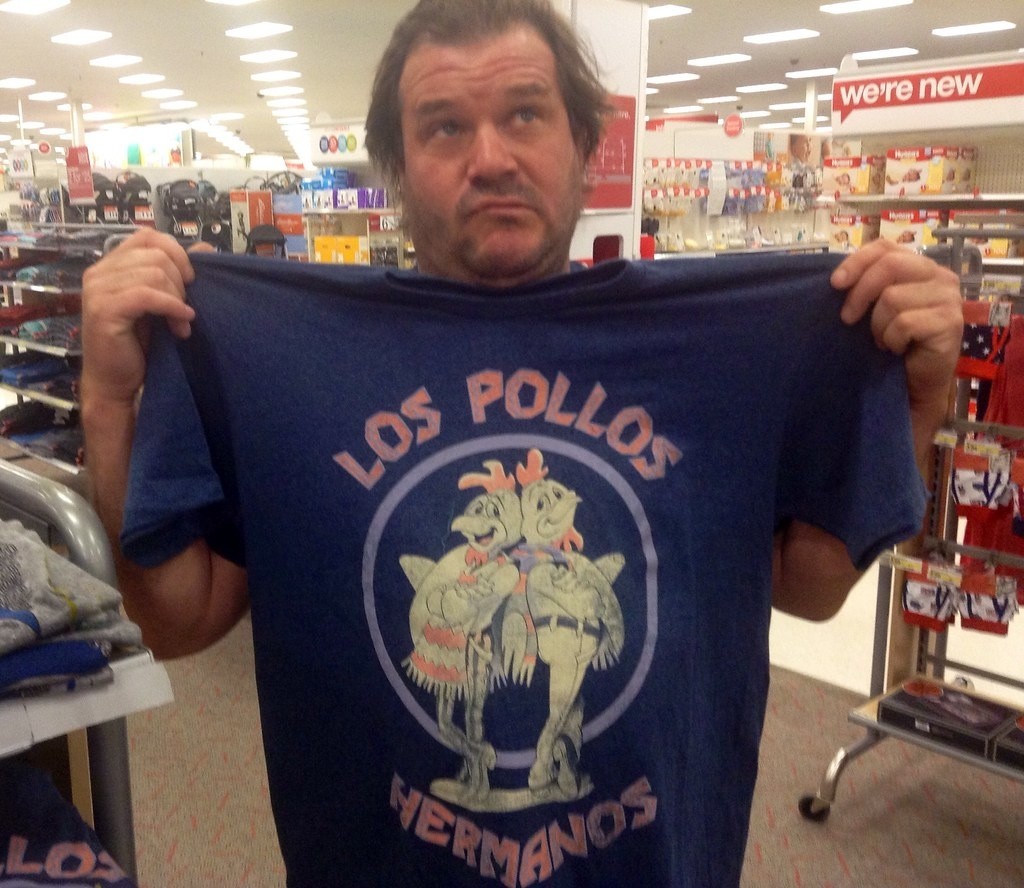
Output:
[790,135,832,166]
[81,1,963,887]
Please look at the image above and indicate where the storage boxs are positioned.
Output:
[822,144,1023,258]
[876,676,1024,773]
[231,167,388,267]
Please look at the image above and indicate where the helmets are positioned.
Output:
[20,172,300,250]
[243,223,287,260]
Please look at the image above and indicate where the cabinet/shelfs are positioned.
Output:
[305,208,405,269]
[0,224,152,478]
[818,193,1023,265]
